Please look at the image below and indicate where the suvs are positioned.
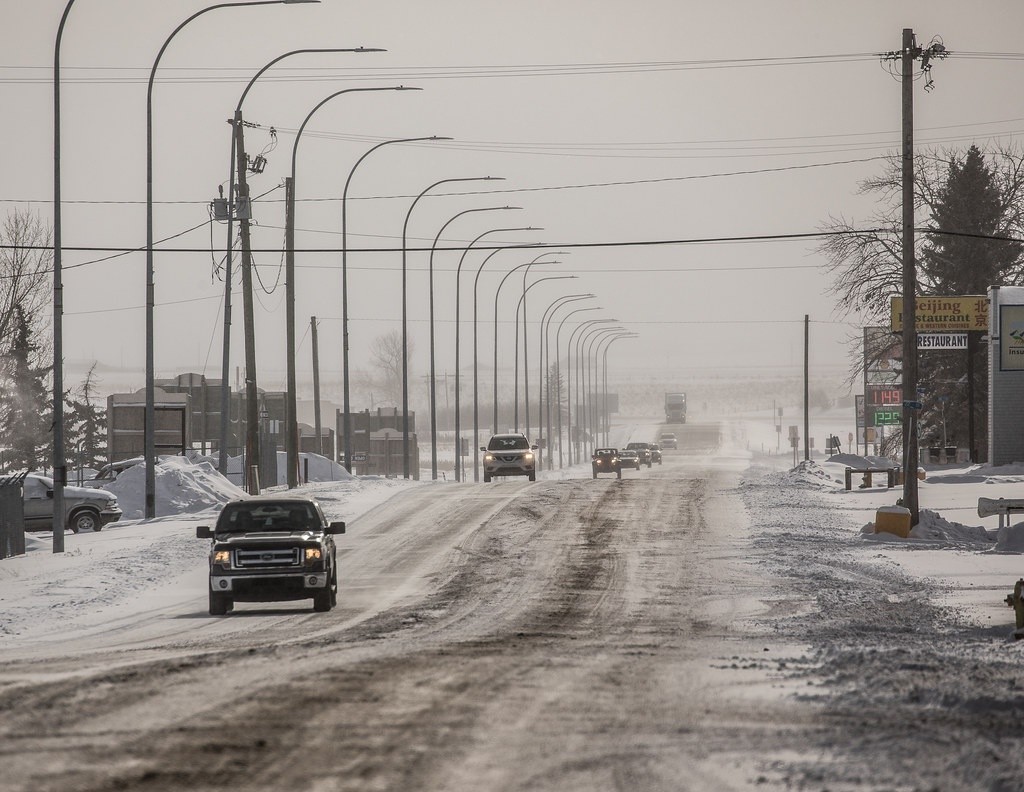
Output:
[195,497,345,612]
[591,448,622,478]
[479,432,538,481]
[622,443,652,468]
[659,433,678,449]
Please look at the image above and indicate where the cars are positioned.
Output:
[618,450,640,470]
[651,444,664,465]
[0,473,122,532]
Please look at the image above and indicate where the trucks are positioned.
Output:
[664,392,687,424]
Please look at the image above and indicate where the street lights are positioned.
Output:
[52,3,645,519]
